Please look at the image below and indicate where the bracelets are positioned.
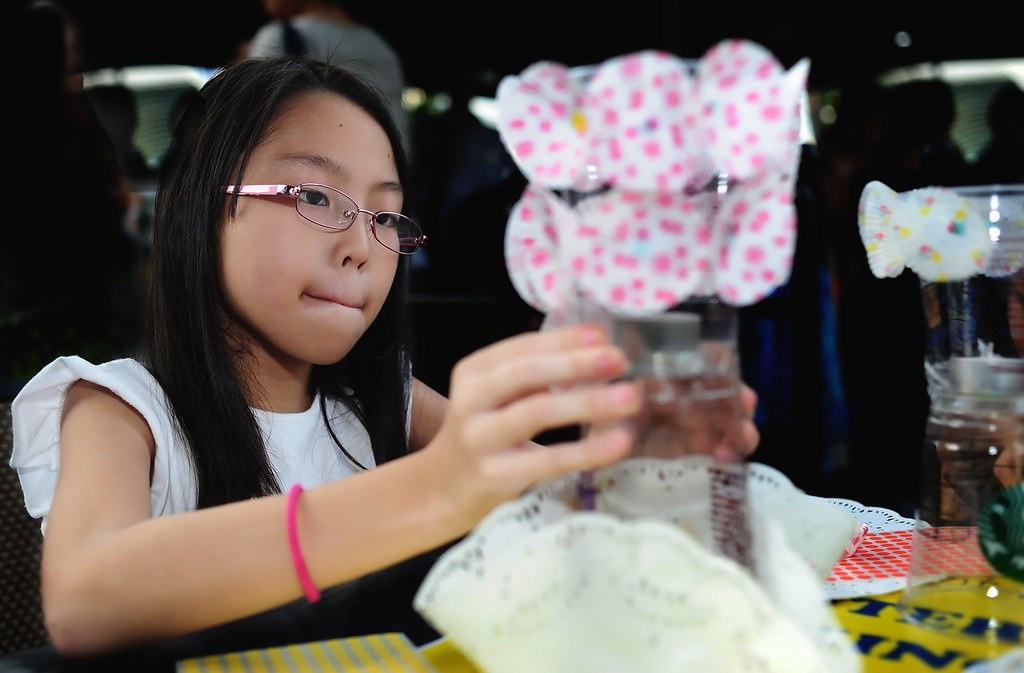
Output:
[287,485,324,604]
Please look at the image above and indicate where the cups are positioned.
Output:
[904,188,1024,673]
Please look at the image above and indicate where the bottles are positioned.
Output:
[576,299,751,567]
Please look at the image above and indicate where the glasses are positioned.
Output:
[218,183,429,257]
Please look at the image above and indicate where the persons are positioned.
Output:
[240,0,411,158]
[8,57,758,655]
[0,6,529,399]
[739,81,1024,517]
